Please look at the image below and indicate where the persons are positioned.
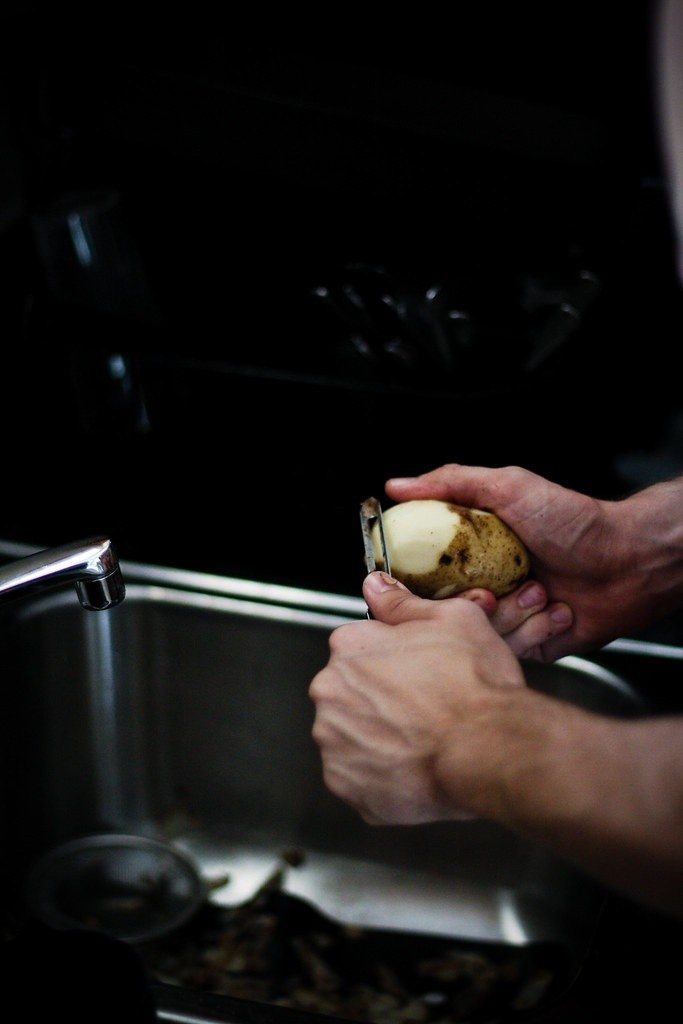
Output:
[308,463,683,920]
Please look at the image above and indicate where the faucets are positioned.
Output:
[0,534,127,612]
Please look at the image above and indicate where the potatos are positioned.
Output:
[369,497,532,600]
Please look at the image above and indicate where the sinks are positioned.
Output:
[0,548,683,1024]
